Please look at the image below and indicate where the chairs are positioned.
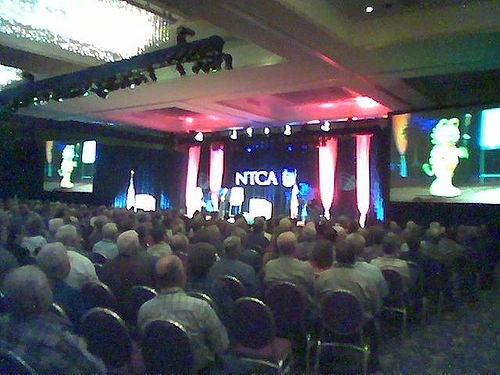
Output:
[51,303,72,325]
[378,268,407,335]
[128,285,158,304]
[81,280,117,309]
[219,275,244,294]
[185,290,219,311]
[408,261,442,322]
[76,307,143,375]
[141,320,193,375]
[313,289,372,374]
[263,283,311,374]
[231,297,295,374]
[0,347,37,375]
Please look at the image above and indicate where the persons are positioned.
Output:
[317,213,381,236]
[264,231,316,300]
[308,237,336,276]
[64,205,107,242]
[171,234,188,265]
[107,206,158,240]
[136,255,230,375]
[24,243,87,321]
[313,236,382,375]
[251,216,317,241]
[370,239,411,300]
[397,232,445,294]
[187,211,250,240]
[185,242,229,309]
[100,230,153,294]
[381,217,419,242]
[158,205,186,239]
[49,225,100,291]
[203,235,259,300]
[343,233,389,314]
[92,222,120,264]
[0,192,64,266]
[429,229,468,285]
[0,264,107,374]
[419,222,453,239]
[144,228,173,257]
